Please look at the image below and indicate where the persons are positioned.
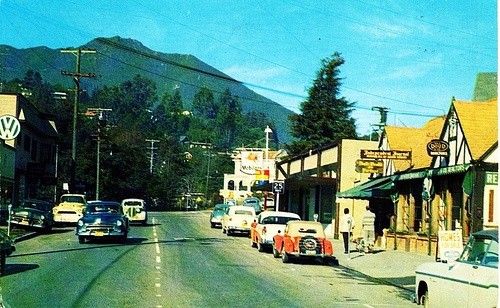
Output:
[362,206,376,252]
[340,208,355,253]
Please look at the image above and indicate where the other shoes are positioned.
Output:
[344,251,348,254]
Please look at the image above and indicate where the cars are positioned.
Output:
[250,211,302,253]
[0,228,16,273]
[221,205,256,235]
[243,198,262,213]
[6,200,55,233]
[272,220,333,263]
[413,230,498,308]
[120,198,148,226]
[75,202,129,243]
[52,193,87,224]
[209,204,231,228]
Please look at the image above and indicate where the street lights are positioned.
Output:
[264,125,274,170]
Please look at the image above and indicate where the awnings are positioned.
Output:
[336,173,397,199]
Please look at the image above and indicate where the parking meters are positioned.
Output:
[7,205,12,236]
[347,219,350,258]
